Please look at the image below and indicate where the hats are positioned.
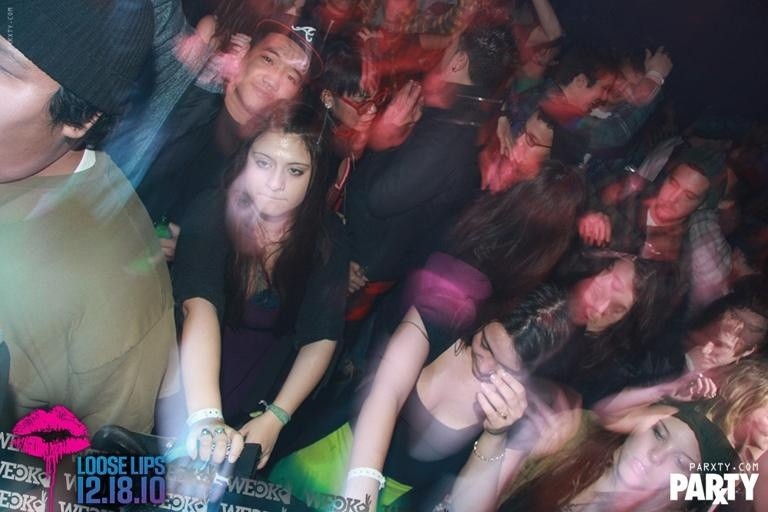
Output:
[0,0,154,115]
[255,12,325,80]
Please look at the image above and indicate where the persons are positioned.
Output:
[0,0,766,512]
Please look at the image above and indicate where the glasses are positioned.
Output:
[517,120,552,150]
[337,85,389,117]
